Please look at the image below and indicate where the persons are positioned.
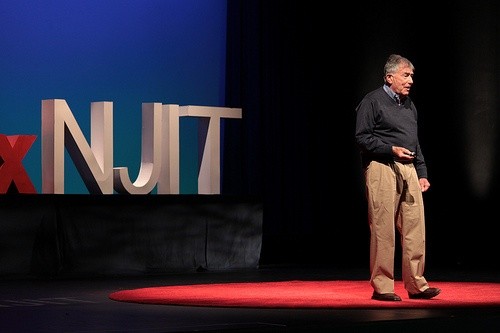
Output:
[355,54,441,301]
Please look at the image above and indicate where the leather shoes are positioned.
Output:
[407,287,441,299]
[370,289,403,301]
